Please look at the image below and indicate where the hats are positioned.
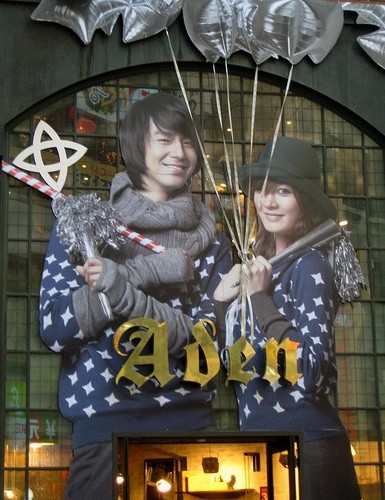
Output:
[240,136,339,221]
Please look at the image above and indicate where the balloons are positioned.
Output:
[30,0,346,68]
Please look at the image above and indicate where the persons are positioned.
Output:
[211,134,361,500]
[37,93,238,499]
